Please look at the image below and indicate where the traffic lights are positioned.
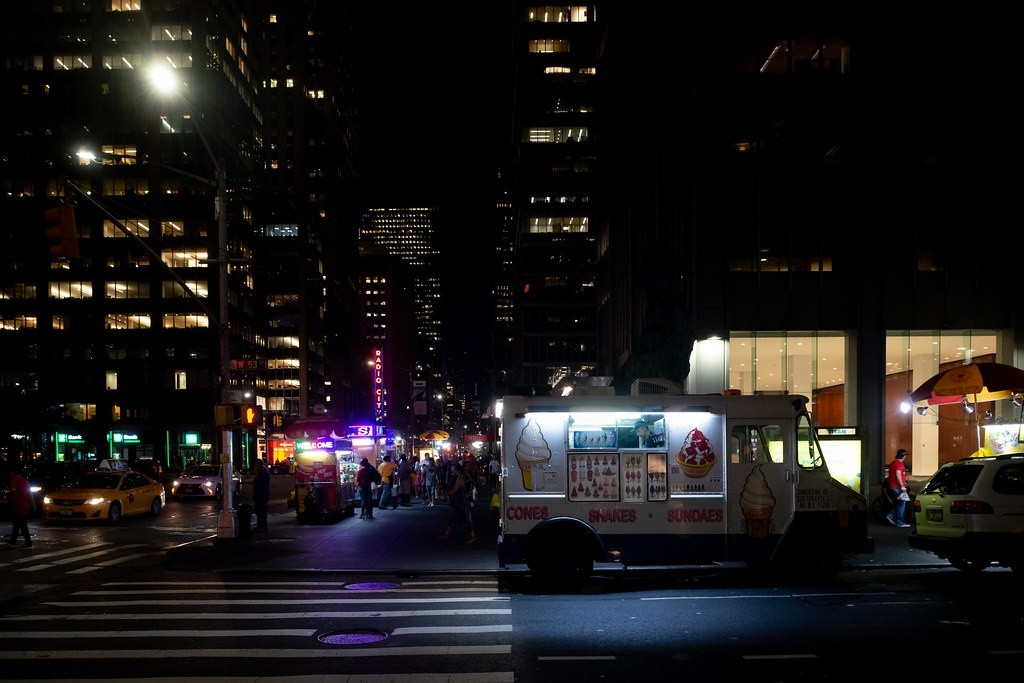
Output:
[242,405,262,426]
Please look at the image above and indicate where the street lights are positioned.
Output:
[74,58,236,540]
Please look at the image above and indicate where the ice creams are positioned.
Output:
[571,431,617,498]
[625,454,665,500]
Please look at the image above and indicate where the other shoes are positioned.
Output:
[414,496,445,507]
[401,503,413,507]
[464,535,478,546]
[4,537,17,544]
[22,541,33,548]
[379,506,389,510]
[393,504,400,509]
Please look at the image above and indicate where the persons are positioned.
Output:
[354,457,381,519]
[412,450,499,521]
[631,421,660,448]
[244,459,271,531]
[437,463,477,544]
[6,466,33,548]
[881,449,911,528]
[377,454,412,509]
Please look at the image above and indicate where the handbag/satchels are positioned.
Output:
[897,488,911,501]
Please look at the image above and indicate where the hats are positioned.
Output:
[897,449,909,455]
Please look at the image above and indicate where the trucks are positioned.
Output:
[492,388,876,584]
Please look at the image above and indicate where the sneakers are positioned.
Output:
[359,512,375,520]
[898,523,911,527]
[886,516,896,525]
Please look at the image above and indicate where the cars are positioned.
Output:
[171,463,242,501]
[0,463,86,515]
[41,459,165,524]
[125,457,171,488]
[269,463,290,475]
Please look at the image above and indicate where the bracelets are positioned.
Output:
[901,485,904,486]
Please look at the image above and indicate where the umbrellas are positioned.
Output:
[283,415,349,450]
[420,430,449,447]
[909,362,1024,451]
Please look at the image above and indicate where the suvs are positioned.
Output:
[907,453,1024,570]
